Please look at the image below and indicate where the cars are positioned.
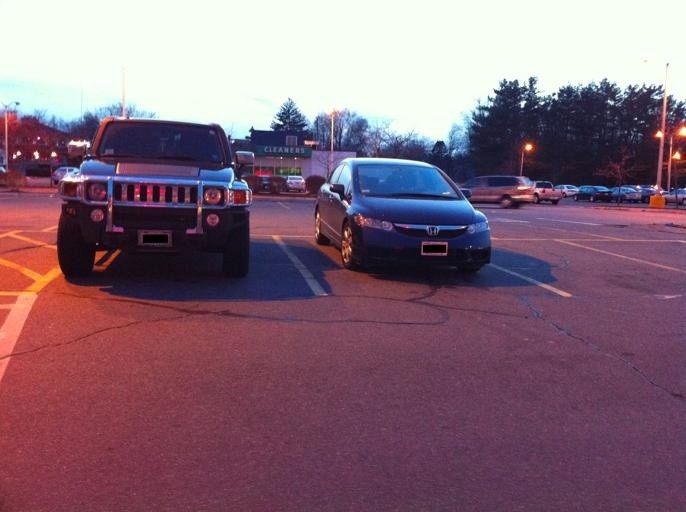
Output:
[459,175,686,208]
[313,158,491,274]
[255,175,307,194]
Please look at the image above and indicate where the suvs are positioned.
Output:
[52,118,254,279]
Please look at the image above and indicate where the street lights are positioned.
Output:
[668,128,686,190]
[328,101,339,170]
[520,144,532,176]
